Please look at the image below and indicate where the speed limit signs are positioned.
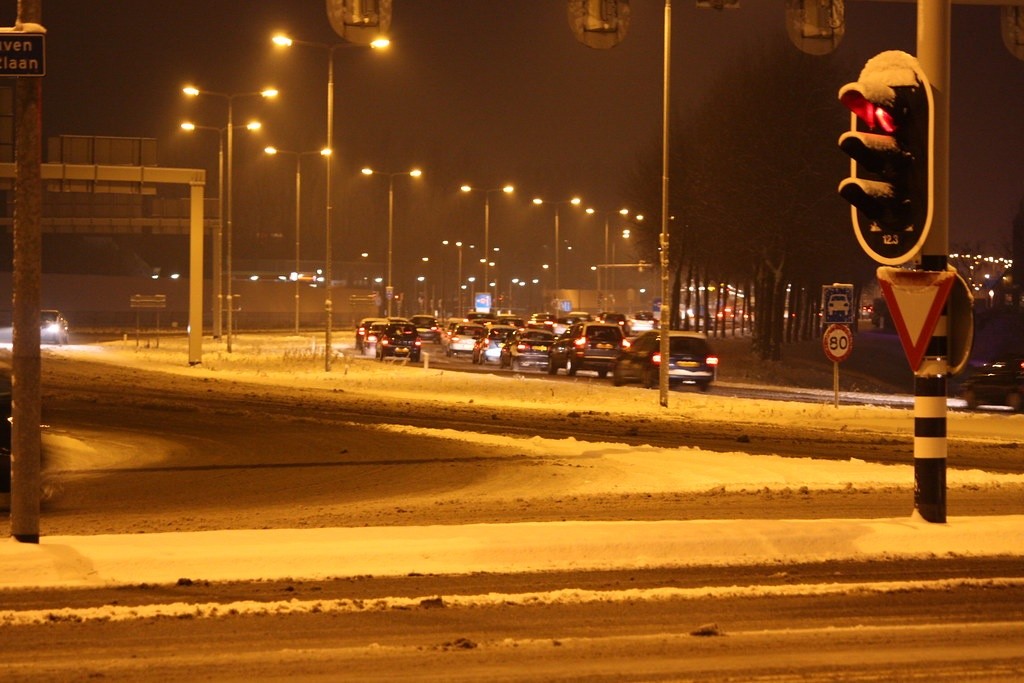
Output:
[822,324,852,361]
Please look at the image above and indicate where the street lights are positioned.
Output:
[586,208,629,312]
[271,35,392,370]
[361,168,422,318]
[183,87,278,350]
[461,186,514,292]
[533,198,581,318]
[265,146,333,337]
[181,122,262,341]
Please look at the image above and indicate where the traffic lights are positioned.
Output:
[835,49,934,264]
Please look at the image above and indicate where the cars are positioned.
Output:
[613,330,720,391]
[472,325,519,364]
[547,322,630,378]
[375,323,421,362]
[354,307,754,359]
[500,328,556,371]
